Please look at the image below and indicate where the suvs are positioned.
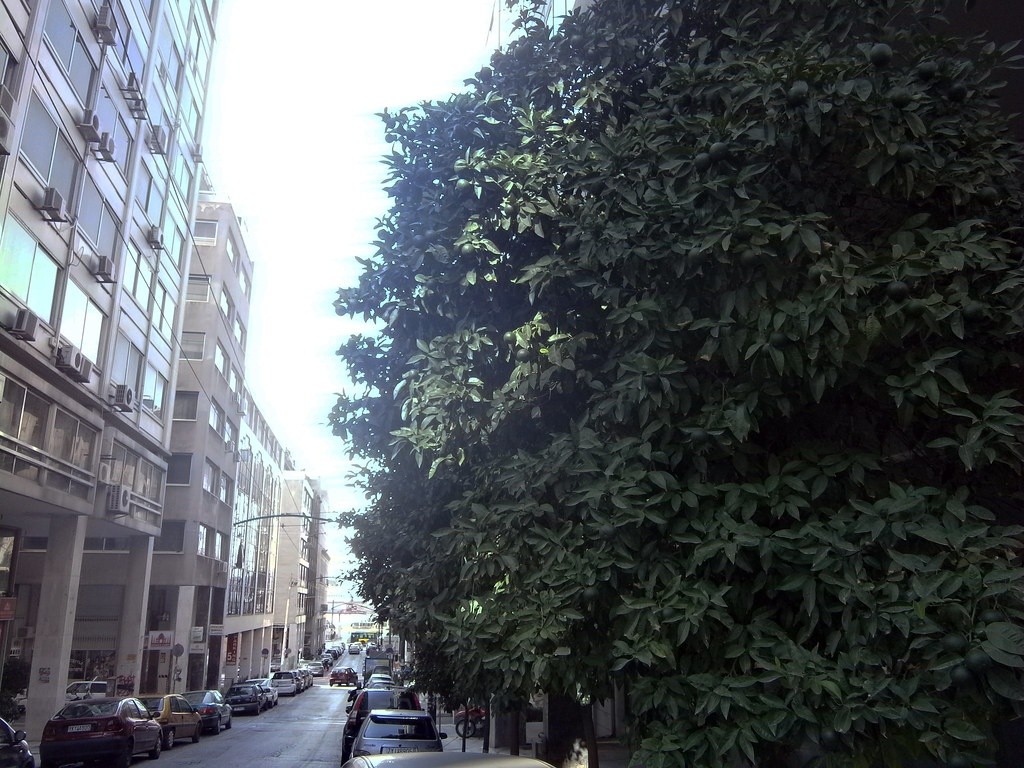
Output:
[339,685,425,768]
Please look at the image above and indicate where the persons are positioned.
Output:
[348,682,365,705]
[322,643,326,651]
[317,647,322,658]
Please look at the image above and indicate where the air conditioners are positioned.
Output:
[106,484,131,514]
[79,69,166,163]
[12,308,39,342]
[96,462,110,485]
[96,5,117,45]
[114,384,134,412]
[233,392,248,416]
[56,345,94,383]
[219,562,227,572]
[44,188,66,220]
[226,441,241,461]
[152,227,164,248]
[99,255,115,283]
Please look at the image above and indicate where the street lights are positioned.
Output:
[278,579,299,671]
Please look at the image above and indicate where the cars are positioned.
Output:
[38,696,164,768]
[179,690,233,735]
[224,641,398,716]
[345,709,448,760]
[128,694,203,751]
[329,667,359,686]
[453,699,534,739]
[339,750,557,768]
[0,716,35,768]
[65,680,108,702]
